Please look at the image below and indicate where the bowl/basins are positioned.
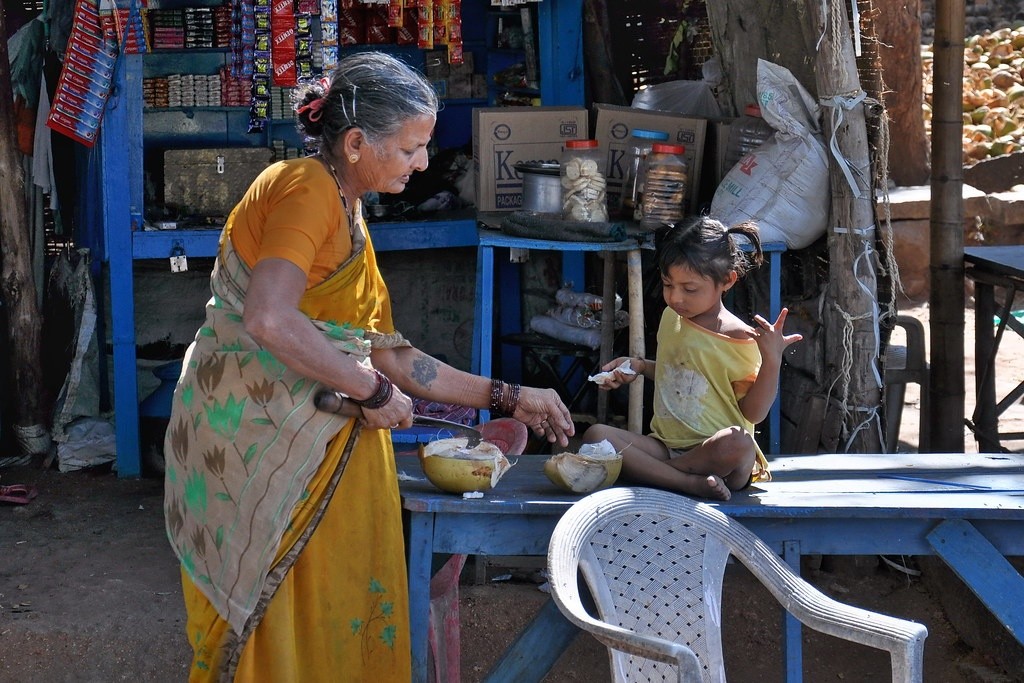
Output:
[366,204,396,218]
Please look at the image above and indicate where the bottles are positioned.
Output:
[640,142,688,228]
[720,104,777,180]
[618,129,670,223]
[563,140,609,223]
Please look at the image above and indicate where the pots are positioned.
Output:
[512,162,563,218]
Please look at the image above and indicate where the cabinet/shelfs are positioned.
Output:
[78,0,586,477]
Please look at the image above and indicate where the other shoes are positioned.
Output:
[0,484,29,504]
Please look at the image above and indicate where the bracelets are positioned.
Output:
[359,371,395,410]
[489,379,521,418]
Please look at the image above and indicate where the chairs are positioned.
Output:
[883,316,930,454]
[500,263,662,455]
[547,487,928,683]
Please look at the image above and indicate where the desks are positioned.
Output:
[395,452,1024,683]
[479,224,788,453]
[964,245,1024,453]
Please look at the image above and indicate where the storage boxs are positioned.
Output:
[163,149,271,218]
[707,117,735,203]
[471,107,588,213]
[590,102,707,215]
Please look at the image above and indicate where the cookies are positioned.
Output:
[562,158,607,222]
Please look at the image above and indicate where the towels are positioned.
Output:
[32,69,58,211]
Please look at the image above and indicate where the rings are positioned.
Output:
[390,424,399,429]
[541,420,546,425]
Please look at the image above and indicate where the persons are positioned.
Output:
[164,50,574,683]
[583,214,803,500]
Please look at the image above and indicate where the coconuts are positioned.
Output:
[545,439,622,495]
[418,437,512,499]
